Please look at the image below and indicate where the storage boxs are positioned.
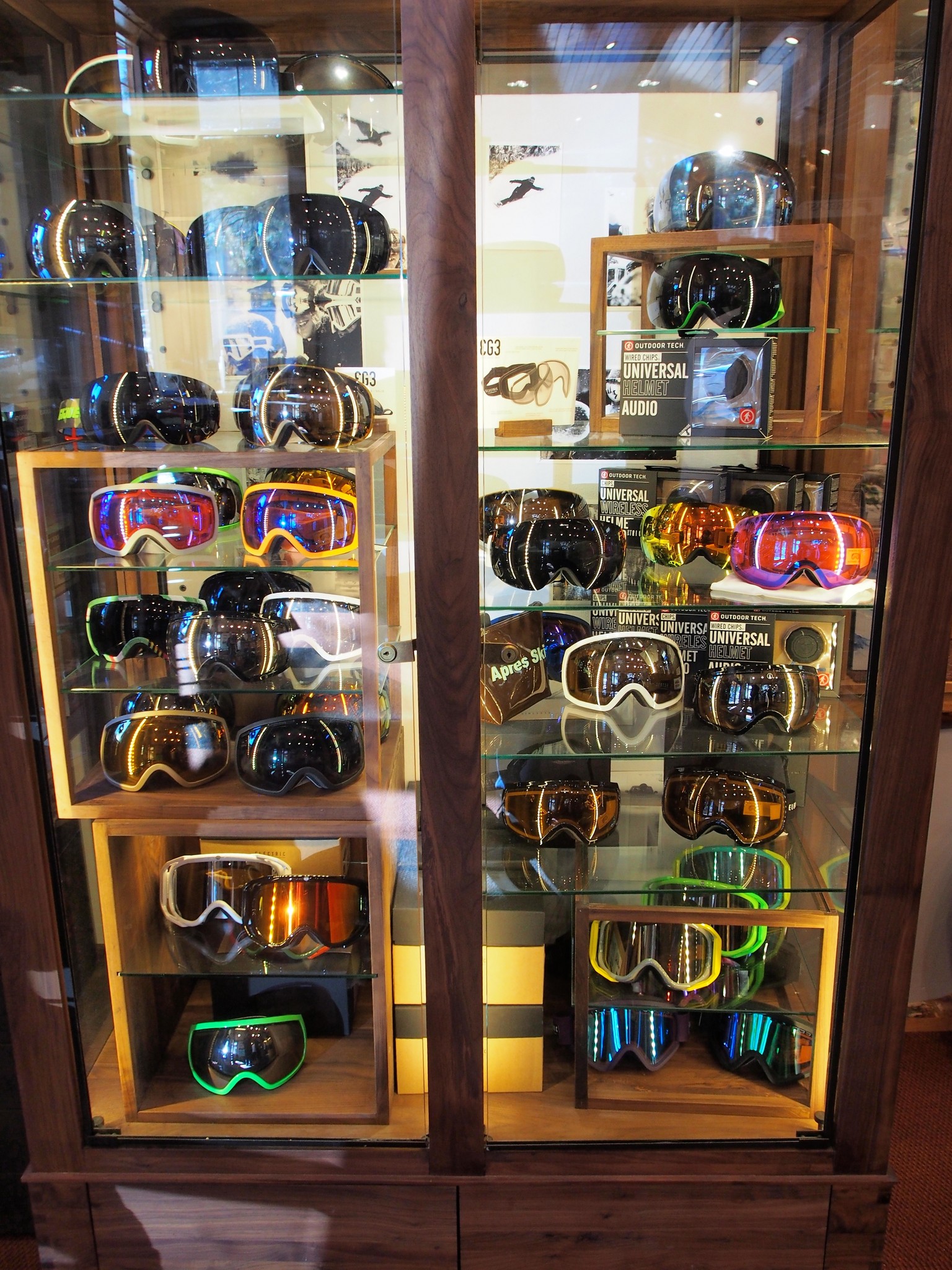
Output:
[589,466,868,701]
[393,777,551,1093]
[212,944,362,1034]
[198,836,352,878]
[619,339,778,441]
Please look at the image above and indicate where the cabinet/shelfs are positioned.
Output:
[572,814,841,1117]
[90,728,406,1125]
[0,0,952,1270]
[14,429,403,820]
[589,223,856,438]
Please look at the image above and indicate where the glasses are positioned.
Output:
[676,849,794,909]
[159,854,294,928]
[232,367,375,450]
[187,1014,308,1096]
[476,489,875,592]
[500,780,622,846]
[653,149,799,233]
[645,249,784,331]
[78,470,366,793]
[640,877,769,960]
[586,1001,685,1069]
[280,50,394,92]
[79,372,221,449]
[242,875,368,951]
[23,191,392,280]
[662,766,788,848]
[488,613,820,738]
[591,921,721,991]
[702,997,814,1086]
